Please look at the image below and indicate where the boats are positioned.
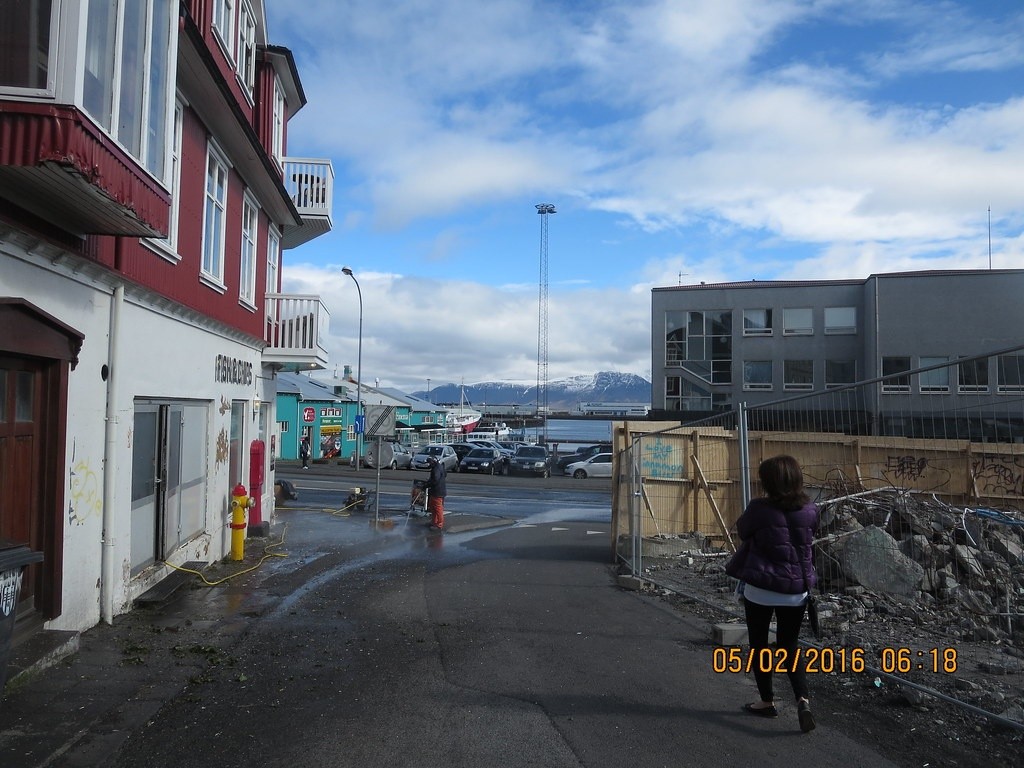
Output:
[443,380,482,433]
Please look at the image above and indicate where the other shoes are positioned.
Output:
[798,700,816,732]
[302,466,308,469]
[426,522,443,531]
[741,702,778,719]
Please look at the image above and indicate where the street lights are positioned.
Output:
[341,266,362,472]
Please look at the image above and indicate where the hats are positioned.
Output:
[426,455,438,463]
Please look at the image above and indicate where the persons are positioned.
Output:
[725,455,820,731]
[300,437,310,470]
[421,455,448,531]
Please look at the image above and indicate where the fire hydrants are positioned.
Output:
[229,482,255,559]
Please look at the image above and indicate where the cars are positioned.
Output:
[508,446,552,478]
[558,443,612,478]
[363,443,412,469]
[447,440,522,475]
[410,445,459,472]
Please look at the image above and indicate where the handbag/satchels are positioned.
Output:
[808,596,820,631]
